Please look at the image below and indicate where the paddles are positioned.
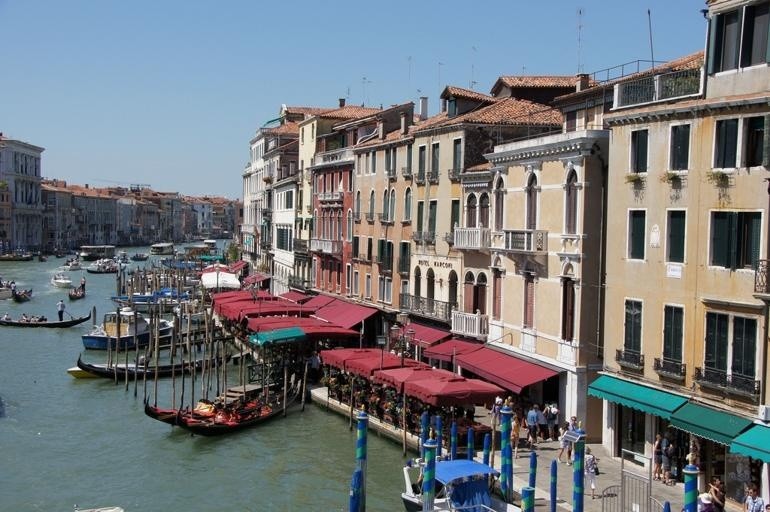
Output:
[18,291,32,301]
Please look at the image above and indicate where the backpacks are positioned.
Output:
[548,407,557,420]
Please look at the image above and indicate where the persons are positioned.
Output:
[3,312,12,322]
[301,342,316,378]
[485,394,586,466]
[584,444,598,500]
[80,275,87,294]
[55,298,66,322]
[70,287,84,298]
[0,277,29,297]
[308,350,322,386]
[650,429,770,512]
[19,313,48,323]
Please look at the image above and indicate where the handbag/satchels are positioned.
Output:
[593,464,599,475]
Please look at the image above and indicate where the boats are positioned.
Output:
[143,373,300,437]
[400,460,500,512]
[80,246,116,261]
[108,269,241,315]
[204,239,218,254]
[150,242,175,255]
[77,351,233,381]
[161,257,209,270]
[130,253,149,262]
[175,244,225,264]
[81,305,175,352]
[112,251,129,264]
[87,258,126,274]
[67,362,138,379]
[0,253,91,328]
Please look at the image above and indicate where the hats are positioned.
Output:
[700,492,712,504]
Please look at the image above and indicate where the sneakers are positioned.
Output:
[558,457,571,466]
